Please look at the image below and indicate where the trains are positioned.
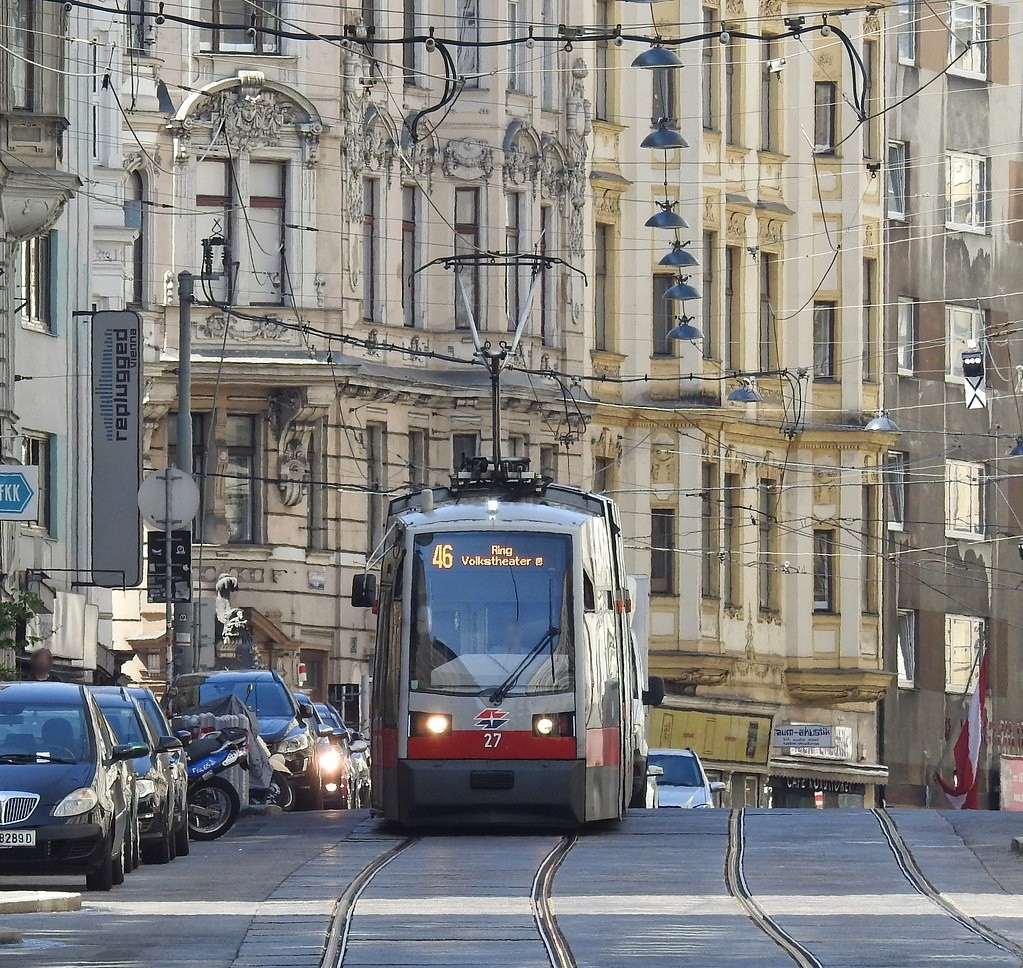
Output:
[351,255,650,834]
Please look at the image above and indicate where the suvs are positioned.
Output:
[159,665,322,812]
[646,745,726,809]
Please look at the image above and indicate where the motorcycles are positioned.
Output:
[173,725,248,843]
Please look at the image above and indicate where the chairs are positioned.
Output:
[5,734,35,753]
[105,715,127,744]
[41,718,86,759]
[487,634,543,654]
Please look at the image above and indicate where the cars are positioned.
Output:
[291,692,373,809]
[78,685,184,865]
[0,682,135,893]
[119,688,194,857]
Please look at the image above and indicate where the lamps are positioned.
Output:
[645,199,690,230]
[640,117,690,150]
[662,275,702,301]
[861,416,900,432]
[665,318,705,340]
[726,385,758,402]
[630,35,685,69]
[658,240,700,267]
[1009,440,1023,455]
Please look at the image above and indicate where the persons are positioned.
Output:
[489,622,535,655]
